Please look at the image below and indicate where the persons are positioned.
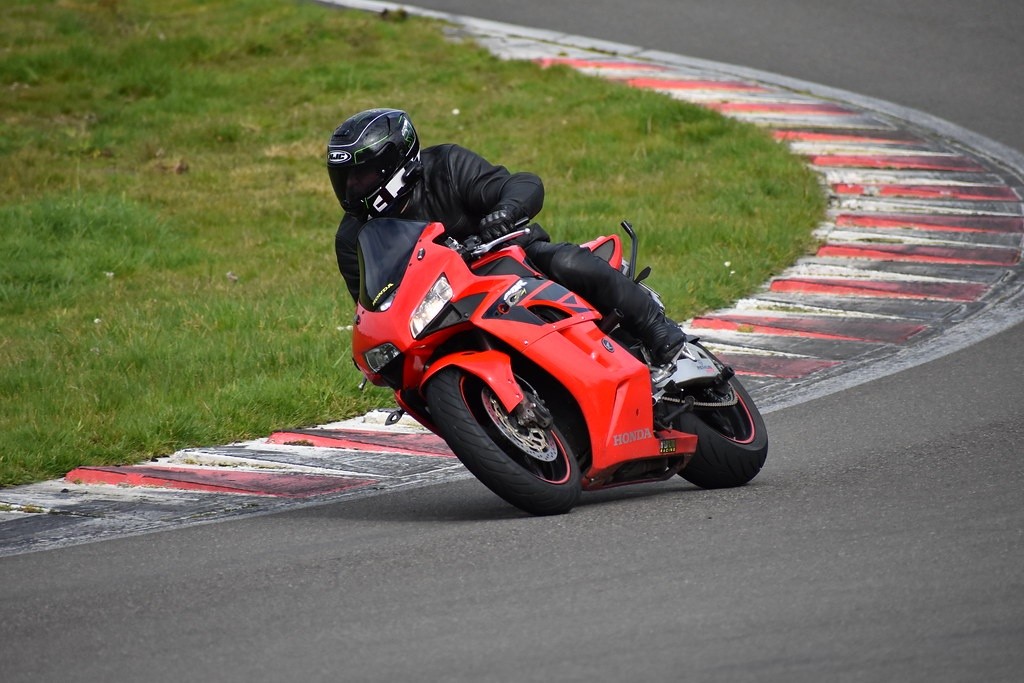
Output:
[327,109,685,366]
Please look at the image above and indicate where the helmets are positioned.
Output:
[327,107,422,221]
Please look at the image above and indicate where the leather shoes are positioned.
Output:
[643,311,688,363]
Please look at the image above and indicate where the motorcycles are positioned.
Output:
[351,214,769,517]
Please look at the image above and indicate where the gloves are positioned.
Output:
[481,205,516,242]
